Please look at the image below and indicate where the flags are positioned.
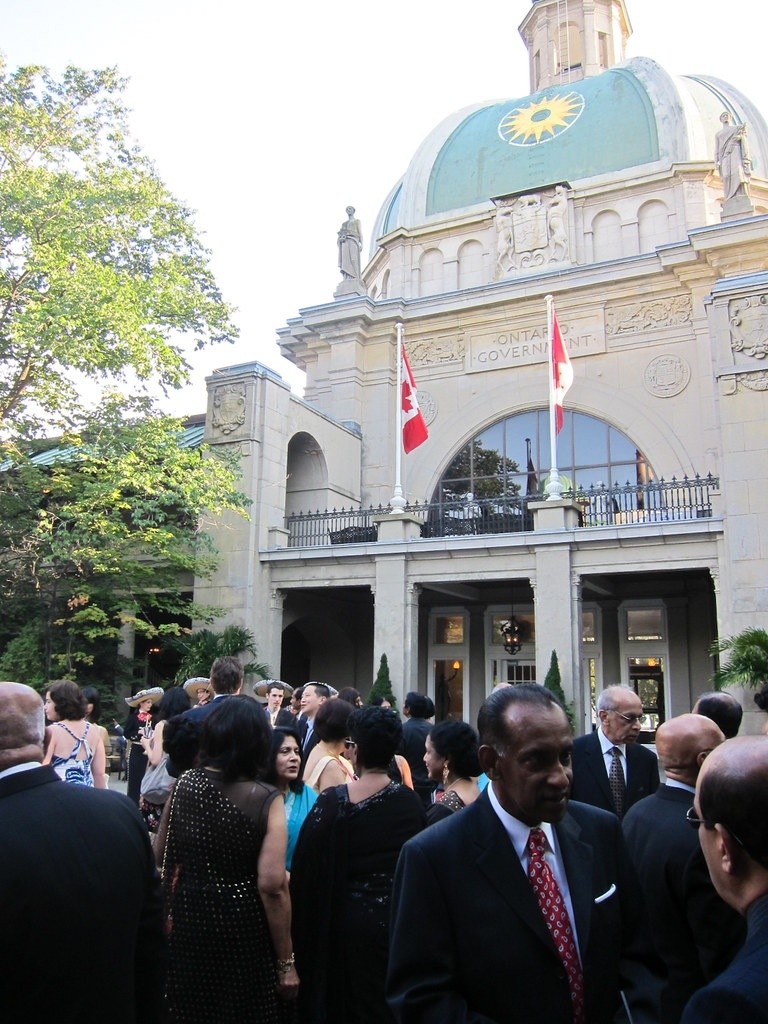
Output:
[401,347,427,454]
[552,312,574,434]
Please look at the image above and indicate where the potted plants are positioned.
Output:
[705,628,768,714]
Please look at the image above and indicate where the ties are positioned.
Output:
[525,827,586,1024]
[606,746,625,819]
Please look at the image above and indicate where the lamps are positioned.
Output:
[648,659,665,729]
[500,584,523,655]
[439,661,461,716]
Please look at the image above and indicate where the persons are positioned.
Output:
[81,685,113,754]
[155,695,300,1024]
[622,713,747,1024]
[44,682,106,790]
[337,206,363,279]
[387,686,665,1024]
[691,690,743,740]
[569,690,660,817]
[0,681,166,1024]
[125,657,512,889]
[715,112,754,201]
[683,735,768,1024]
[289,708,427,1024]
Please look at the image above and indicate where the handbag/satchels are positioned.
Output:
[139,719,178,805]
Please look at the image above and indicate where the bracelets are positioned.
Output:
[279,953,295,972]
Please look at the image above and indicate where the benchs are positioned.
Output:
[329,513,536,544]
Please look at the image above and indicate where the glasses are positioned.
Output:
[343,736,356,749]
[606,709,647,724]
[686,807,743,849]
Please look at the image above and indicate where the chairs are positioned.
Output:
[105,737,128,780]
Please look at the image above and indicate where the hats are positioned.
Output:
[183,676,215,700]
[124,686,164,708]
[253,679,294,699]
[303,681,339,699]
